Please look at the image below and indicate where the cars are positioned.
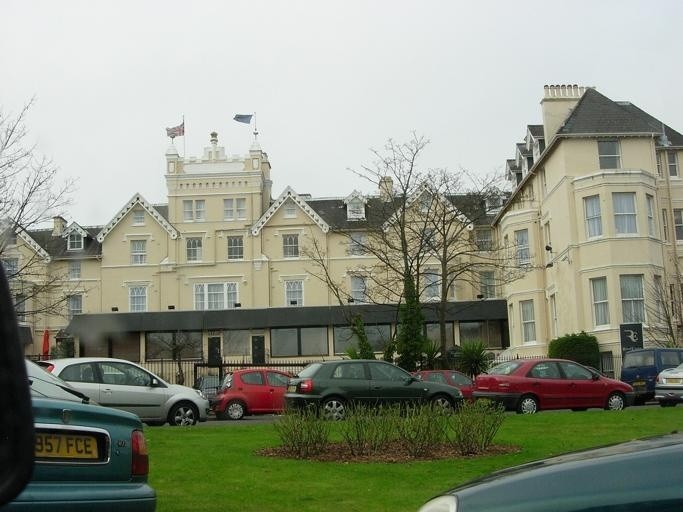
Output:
[31,358,212,428]
[470,358,639,415]
[208,368,297,419]
[283,358,464,422]
[418,433,682,512]
[653,362,683,407]
[0,357,157,510]
[409,370,473,406]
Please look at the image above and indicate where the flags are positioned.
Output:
[233,113,254,126]
[165,113,184,138]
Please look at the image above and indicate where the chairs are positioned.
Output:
[97,368,105,382]
[82,368,93,381]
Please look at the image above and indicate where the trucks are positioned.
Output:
[620,347,683,406]
